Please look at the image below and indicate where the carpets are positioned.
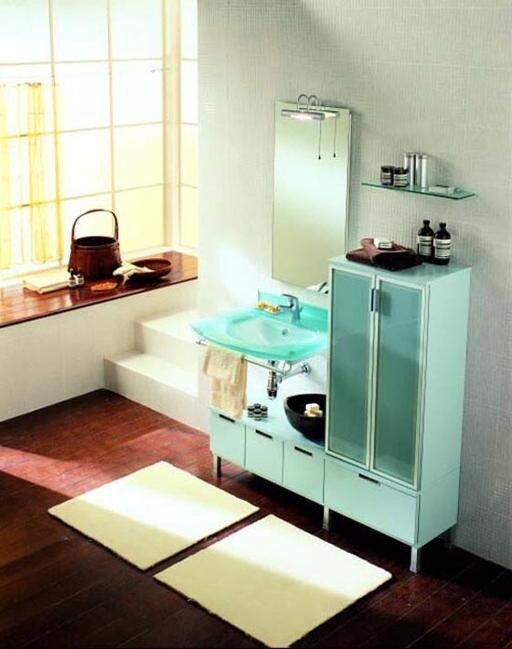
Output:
[153,510,394,648]
[50,463,260,569]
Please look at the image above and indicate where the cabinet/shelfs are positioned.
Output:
[213,396,470,572]
[325,254,472,492]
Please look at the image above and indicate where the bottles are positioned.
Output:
[67,266,85,288]
[380,152,430,189]
[417,220,452,265]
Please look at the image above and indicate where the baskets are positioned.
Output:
[68,209,172,279]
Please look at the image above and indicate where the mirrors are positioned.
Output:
[270,101,351,293]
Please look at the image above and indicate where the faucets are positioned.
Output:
[279,293,304,322]
[318,279,328,293]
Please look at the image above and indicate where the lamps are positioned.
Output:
[277,91,332,160]
[301,108,340,158]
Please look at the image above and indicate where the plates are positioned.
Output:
[125,259,172,280]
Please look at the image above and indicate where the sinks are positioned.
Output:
[224,307,328,351]
